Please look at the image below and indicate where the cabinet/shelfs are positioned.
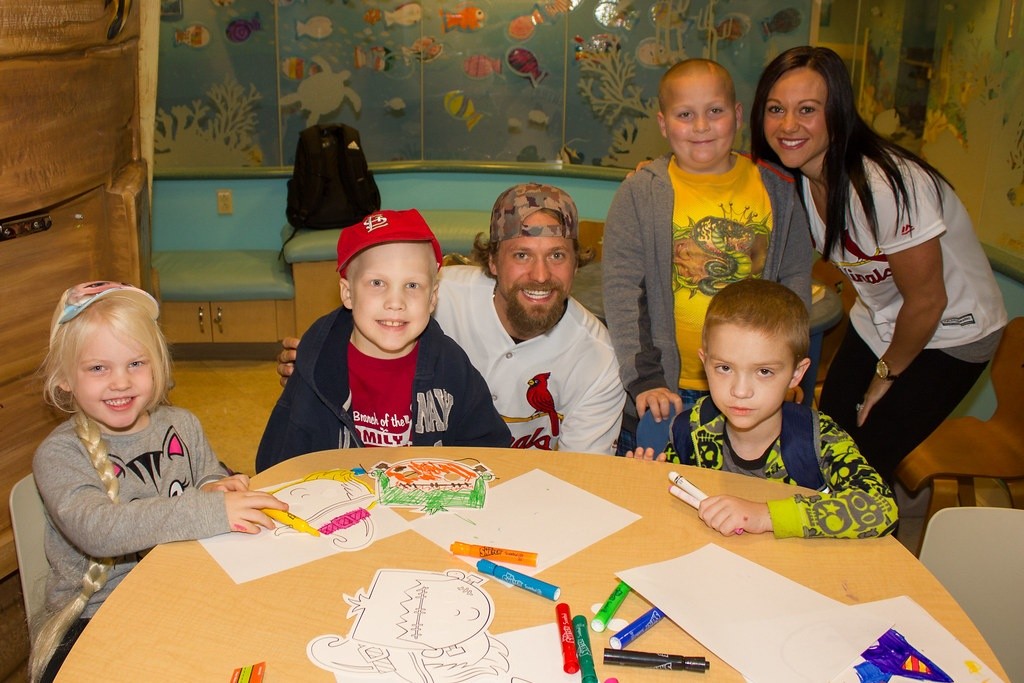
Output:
[152,250,295,343]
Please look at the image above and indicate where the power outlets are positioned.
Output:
[216,189,233,215]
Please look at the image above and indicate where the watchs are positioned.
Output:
[875,359,898,380]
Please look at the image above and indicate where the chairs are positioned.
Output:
[800,258,1024,683]
[9,473,53,646]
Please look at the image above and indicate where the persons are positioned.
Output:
[625,279,898,538]
[27,282,288,683]
[751,45,1007,542]
[277,184,629,457]
[255,209,511,475]
[600,59,813,456]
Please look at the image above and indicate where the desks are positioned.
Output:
[54,446,1013,683]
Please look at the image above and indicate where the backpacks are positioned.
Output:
[286,124,381,230]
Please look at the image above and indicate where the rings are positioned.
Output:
[855,403,864,411]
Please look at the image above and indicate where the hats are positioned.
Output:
[49,281,160,353]
[335,207,443,279]
[489,182,579,242]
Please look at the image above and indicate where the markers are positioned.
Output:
[258,509,320,539]
[555,603,580,674]
[668,471,709,501]
[590,581,632,633]
[572,616,599,683]
[602,648,710,673]
[476,559,561,602]
[450,542,538,568]
[608,607,666,650]
[668,486,744,535]
